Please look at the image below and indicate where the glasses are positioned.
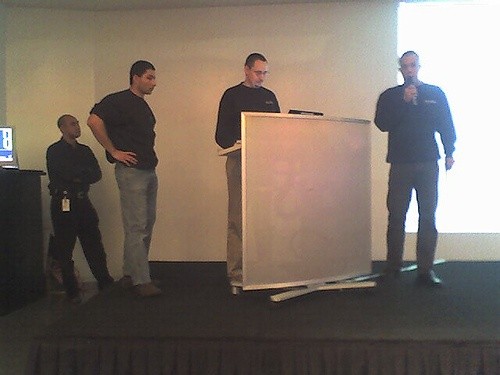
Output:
[252,68,270,76]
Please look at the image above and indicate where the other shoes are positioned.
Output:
[230,275,243,286]
[66,291,82,304]
[416,268,442,286]
[125,271,163,287]
[134,282,162,297]
[379,270,400,280]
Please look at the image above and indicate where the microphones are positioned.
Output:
[407,77,417,106]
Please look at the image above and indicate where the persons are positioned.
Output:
[45,114,125,300]
[373,49,458,287]
[214,52,282,295]
[85,59,163,299]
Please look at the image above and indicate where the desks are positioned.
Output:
[0,166,49,316]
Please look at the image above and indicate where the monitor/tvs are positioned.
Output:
[289,110,323,116]
[0,126,16,166]
